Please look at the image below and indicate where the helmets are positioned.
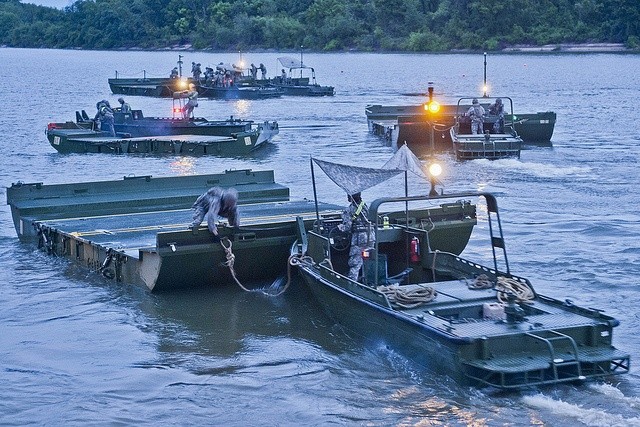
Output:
[225,192,237,206]
[472,99,478,102]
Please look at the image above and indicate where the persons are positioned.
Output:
[487,98,507,134]
[250,64,257,80]
[170,67,178,80]
[182,83,198,121]
[280,68,287,82]
[118,98,132,115]
[93,98,115,136]
[467,99,486,135]
[191,187,240,242]
[256,64,267,80]
[191,62,202,80]
[337,192,375,282]
[203,62,244,88]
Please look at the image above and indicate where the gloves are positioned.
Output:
[215,235,220,242]
[338,223,343,230]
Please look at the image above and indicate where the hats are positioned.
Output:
[496,98,502,104]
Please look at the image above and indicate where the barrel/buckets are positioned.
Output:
[484,301,505,319]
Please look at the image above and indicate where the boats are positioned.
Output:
[289,82,637,391]
[45,90,280,159]
[451,53,521,159]
[246,46,336,97]
[109,73,280,98]
[6,167,349,290]
[365,103,559,154]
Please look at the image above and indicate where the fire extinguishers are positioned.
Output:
[411,236,420,262]
